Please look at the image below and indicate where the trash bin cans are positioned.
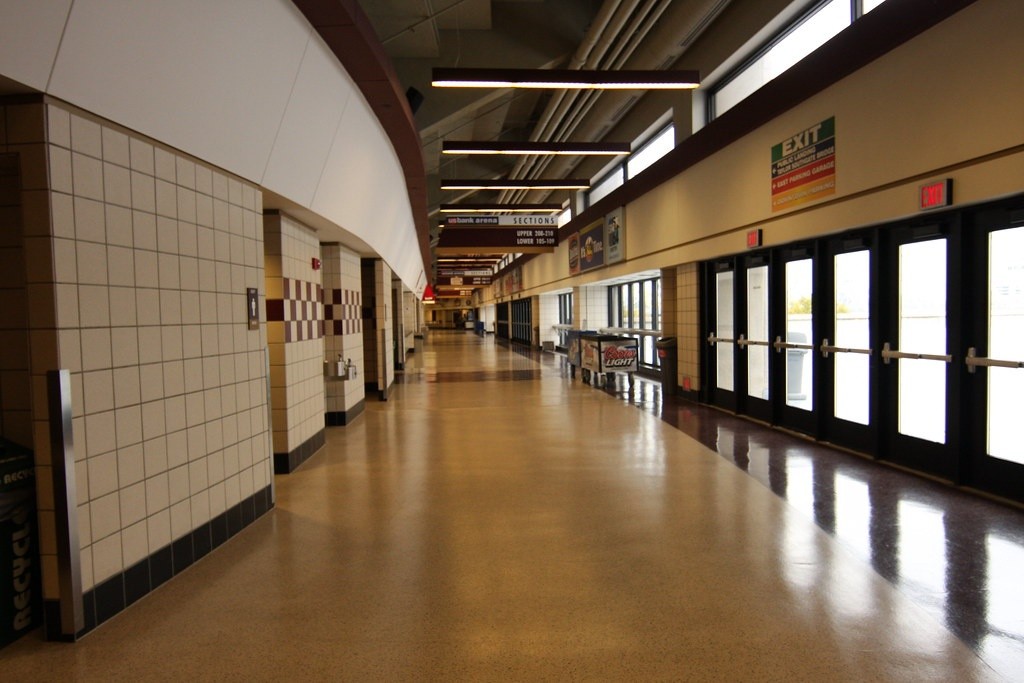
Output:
[654,337,677,394]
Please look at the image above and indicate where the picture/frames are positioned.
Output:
[605,206,627,265]
[580,216,606,274]
[568,232,581,277]
[492,265,523,296]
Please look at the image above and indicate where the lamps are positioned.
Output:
[430,66,701,90]
[440,202,562,213]
[442,139,631,155]
[441,179,591,190]
